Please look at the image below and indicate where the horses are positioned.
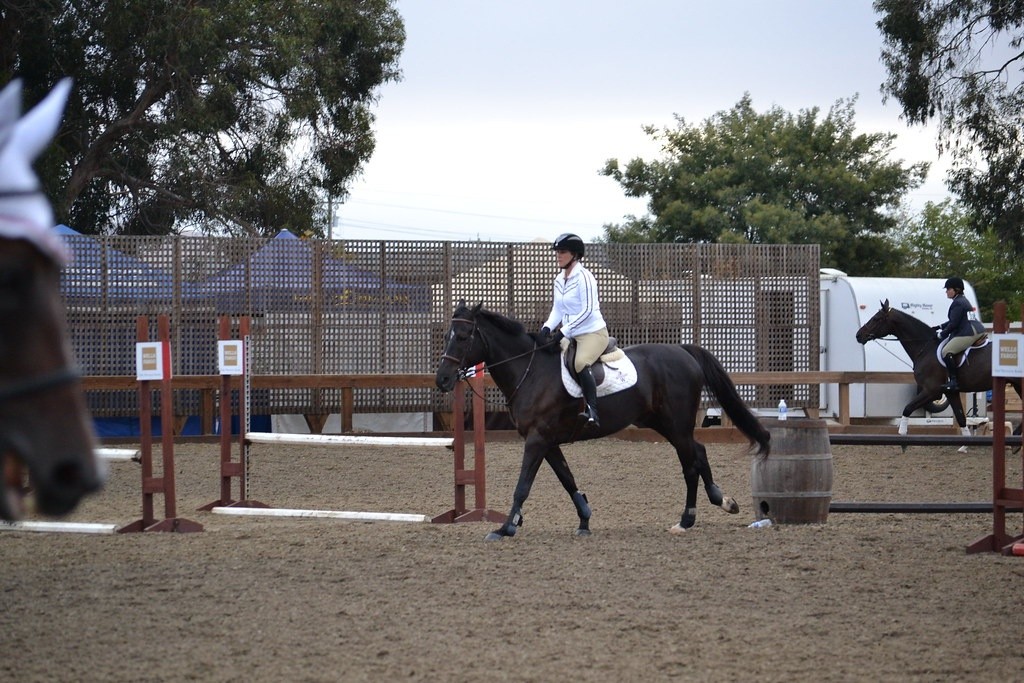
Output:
[1,77,106,527]
[856,298,1024,454]
[434,297,773,543]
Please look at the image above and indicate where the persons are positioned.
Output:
[539,233,609,428]
[931,278,985,391]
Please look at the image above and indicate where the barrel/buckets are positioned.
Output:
[754,418,833,526]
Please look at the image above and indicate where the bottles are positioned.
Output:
[778,400,788,420]
[747,519,772,529]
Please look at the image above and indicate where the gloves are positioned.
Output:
[552,331,565,343]
[540,327,550,337]
[937,332,943,339]
[932,325,940,330]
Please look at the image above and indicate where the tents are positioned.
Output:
[48,229,677,432]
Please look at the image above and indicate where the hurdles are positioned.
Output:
[76,297,1023,556]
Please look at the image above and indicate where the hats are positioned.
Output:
[944,278,964,290]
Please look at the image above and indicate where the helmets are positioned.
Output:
[550,233,585,256]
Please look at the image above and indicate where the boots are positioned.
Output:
[941,354,960,390]
[578,363,601,437]
